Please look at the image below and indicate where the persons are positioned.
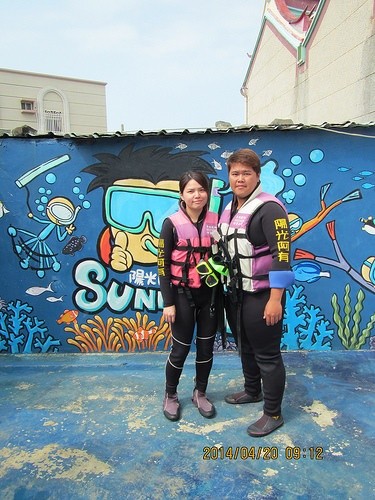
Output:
[218,150,295,437]
[156,171,231,421]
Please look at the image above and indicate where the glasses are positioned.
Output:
[208,257,229,276]
[195,259,218,287]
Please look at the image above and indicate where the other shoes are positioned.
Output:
[247,415,284,437]
[224,391,264,404]
[163,391,179,421]
[193,388,215,417]
